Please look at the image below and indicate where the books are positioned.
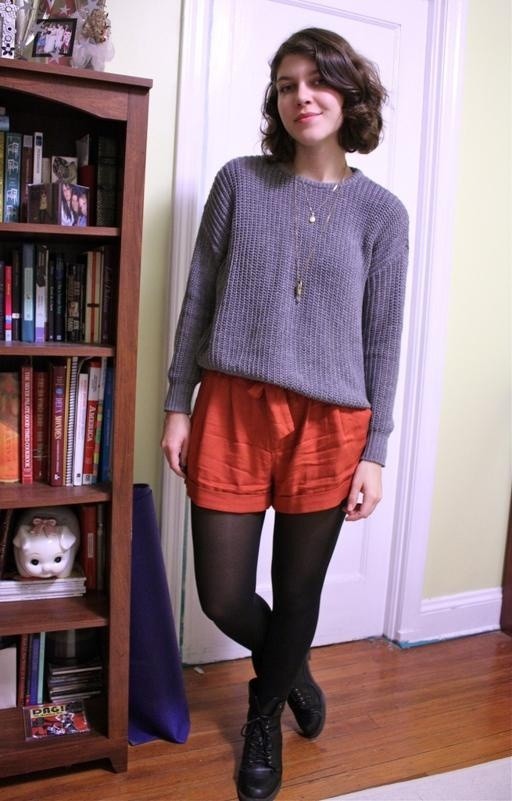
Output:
[0,130,114,226]
[1,628,104,707]
[0,504,110,602]
[0,355,112,487]
[0,243,115,343]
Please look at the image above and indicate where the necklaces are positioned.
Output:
[292,160,349,305]
[292,160,349,224]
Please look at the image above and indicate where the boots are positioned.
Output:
[237,677,283,800]
[286,650,326,737]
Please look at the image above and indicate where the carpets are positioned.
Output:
[318,755,511,801]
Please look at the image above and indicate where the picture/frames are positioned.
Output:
[33,17,78,57]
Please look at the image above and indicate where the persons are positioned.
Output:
[160,28,411,800]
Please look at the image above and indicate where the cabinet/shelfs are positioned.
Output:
[0,55,156,786]
